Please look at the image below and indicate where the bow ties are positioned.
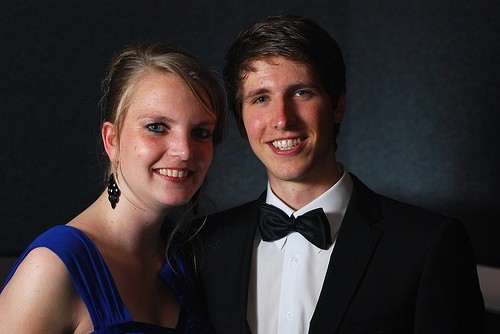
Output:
[255,201,332,251]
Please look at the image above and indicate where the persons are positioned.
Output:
[0,43,228,334]
[178,13,488,334]
